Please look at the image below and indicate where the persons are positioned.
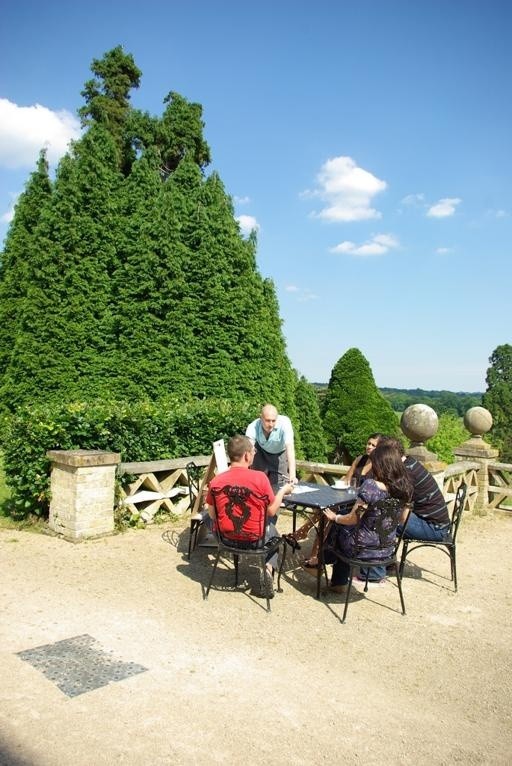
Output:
[353,438,450,582]
[206,434,294,599]
[282,433,384,557]
[245,403,298,523]
[302,446,415,592]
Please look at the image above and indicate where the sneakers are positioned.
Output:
[260,569,274,598]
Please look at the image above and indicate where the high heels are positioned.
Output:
[302,560,323,578]
[330,583,347,592]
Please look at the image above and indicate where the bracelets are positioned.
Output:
[334,513,341,524]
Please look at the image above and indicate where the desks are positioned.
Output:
[271,479,359,600]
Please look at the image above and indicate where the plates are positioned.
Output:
[331,485,349,490]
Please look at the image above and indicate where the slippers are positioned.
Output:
[282,534,301,550]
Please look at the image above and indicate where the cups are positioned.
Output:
[347,478,356,494]
[335,481,345,488]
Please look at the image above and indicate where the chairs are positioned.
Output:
[186,462,287,613]
[311,478,467,624]
